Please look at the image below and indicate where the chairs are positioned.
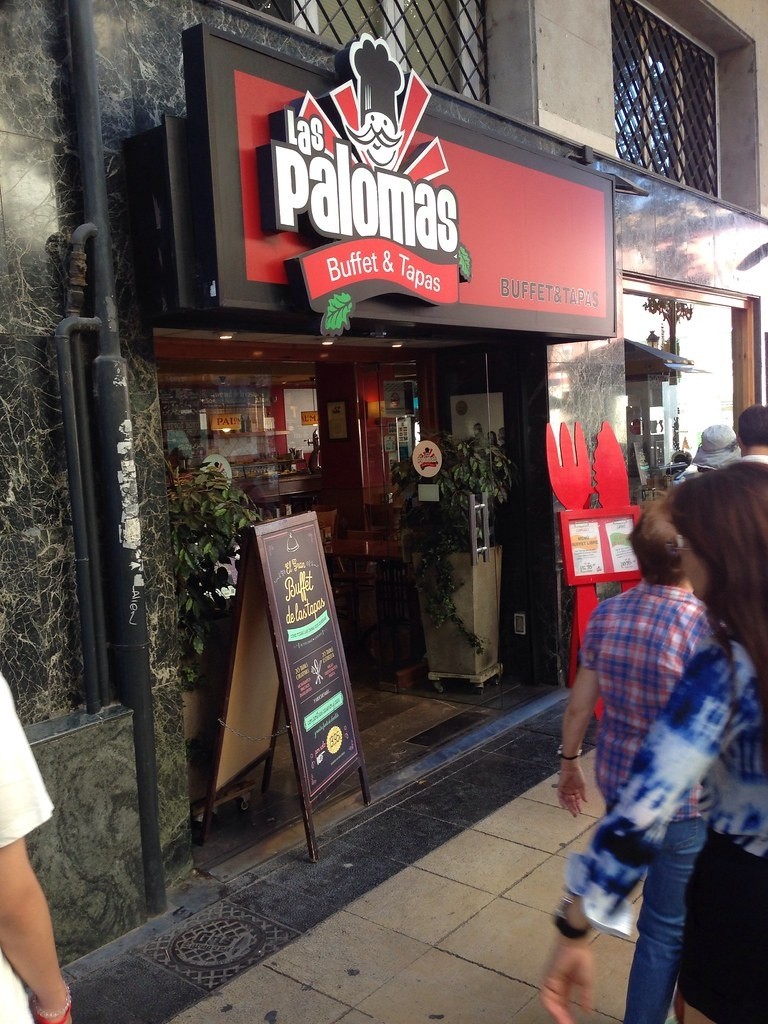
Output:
[313,507,349,575]
[366,503,396,528]
[348,529,389,620]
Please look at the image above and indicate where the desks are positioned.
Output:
[322,537,403,642]
[278,457,308,468]
[236,472,323,502]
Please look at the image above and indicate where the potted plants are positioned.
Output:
[172,469,264,816]
[389,426,517,690]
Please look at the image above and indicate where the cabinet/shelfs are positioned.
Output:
[204,402,290,439]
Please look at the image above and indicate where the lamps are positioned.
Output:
[301,376,318,427]
[209,375,242,433]
[367,363,386,418]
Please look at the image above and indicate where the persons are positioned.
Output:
[539,402,768,1024]
[0,669,76,1024]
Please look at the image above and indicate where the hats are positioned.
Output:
[691,424,741,469]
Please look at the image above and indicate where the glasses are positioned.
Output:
[672,535,691,551]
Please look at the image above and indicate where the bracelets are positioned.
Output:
[557,744,583,760]
[30,979,72,1024]
[553,893,589,940]
